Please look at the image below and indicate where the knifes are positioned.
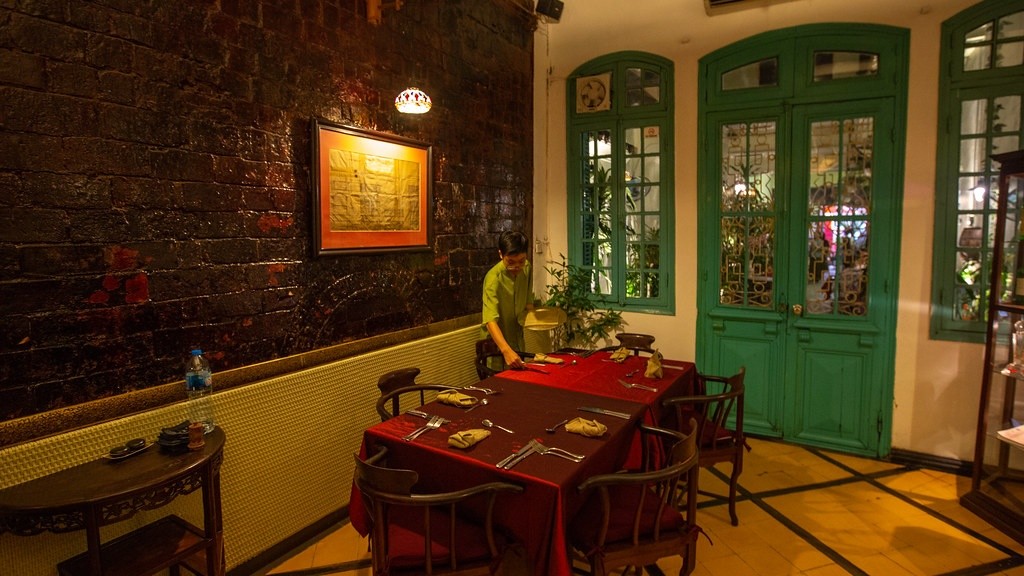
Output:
[522,361,546,368]
[406,410,451,424]
[577,406,631,419]
[496,444,535,470]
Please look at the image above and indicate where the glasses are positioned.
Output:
[503,254,529,270]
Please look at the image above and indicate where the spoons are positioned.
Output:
[482,419,513,434]
[546,420,568,431]
[561,360,576,367]
[465,398,489,413]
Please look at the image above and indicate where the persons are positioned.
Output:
[479,229,534,374]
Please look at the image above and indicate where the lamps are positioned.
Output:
[968,180,986,202]
[394,63,432,114]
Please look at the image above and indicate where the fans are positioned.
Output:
[626,177,651,204]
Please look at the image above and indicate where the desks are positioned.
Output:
[348,348,695,576]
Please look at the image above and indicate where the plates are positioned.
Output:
[103,441,154,459]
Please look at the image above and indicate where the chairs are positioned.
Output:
[376,367,463,422]
[571,419,712,576]
[475,338,535,381]
[598,333,663,360]
[354,444,523,576]
[663,367,751,526]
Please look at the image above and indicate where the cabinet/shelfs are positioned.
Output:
[959,149,1024,546]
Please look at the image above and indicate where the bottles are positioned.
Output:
[186,350,214,434]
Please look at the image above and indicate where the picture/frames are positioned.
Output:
[309,116,433,257]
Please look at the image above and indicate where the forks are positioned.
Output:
[619,379,657,394]
[530,439,585,462]
[403,416,444,441]
